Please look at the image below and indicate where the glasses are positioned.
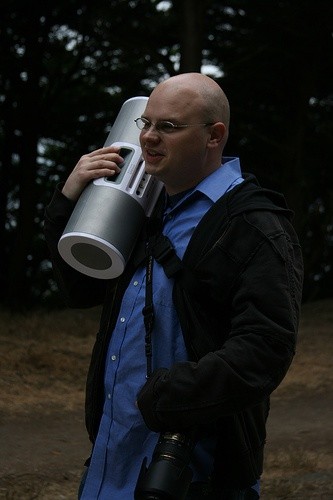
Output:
[134,118,214,133]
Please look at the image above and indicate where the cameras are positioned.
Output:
[134,423,199,500]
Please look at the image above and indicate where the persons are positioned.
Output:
[36,72,304,500]
